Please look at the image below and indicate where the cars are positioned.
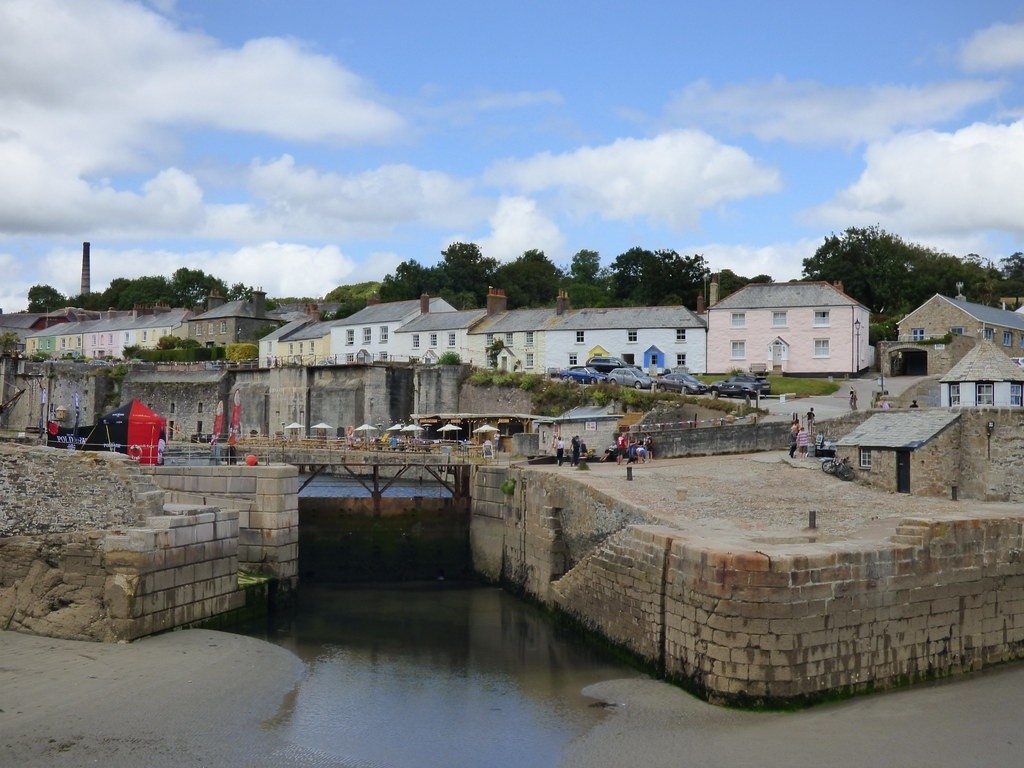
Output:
[88,360,109,365]
[585,356,643,374]
[191,434,226,442]
[559,366,607,385]
[657,372,709,395]
[210,360,237,368]
[131,359,152,365]
[606,368,657,389]
[24,426,45,433]
[317,360,334,365]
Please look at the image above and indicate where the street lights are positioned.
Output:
[854,318,861,378]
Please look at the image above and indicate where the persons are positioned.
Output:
[554,436,565,467]
[849,386,858,410]
[880,396,889,408]
[788,408,815,462]
[493,431,500,452]
[570,435,580,467]
[909,400,919,408]
[597,432,654,465]
[484,437,493,445]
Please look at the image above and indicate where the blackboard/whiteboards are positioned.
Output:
[484,444,493,456]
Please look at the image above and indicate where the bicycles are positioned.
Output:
[822,456,855,481]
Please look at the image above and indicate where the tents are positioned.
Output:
[97,399,167,465]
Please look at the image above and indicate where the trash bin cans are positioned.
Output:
[227,446,237,464]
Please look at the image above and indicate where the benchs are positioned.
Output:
[237,436,497,464]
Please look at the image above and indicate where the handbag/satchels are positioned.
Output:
[605,449,610,454]
[649,443,654,449]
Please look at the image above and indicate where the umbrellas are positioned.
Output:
[436,424,462,440]
[285,422,305,436]
[400,425,425,431]
[354,424,377,444]
[310,423,333,436]
[472,425,500,441]
[386,424,406,430]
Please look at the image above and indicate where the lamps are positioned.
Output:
[984,420,995,435]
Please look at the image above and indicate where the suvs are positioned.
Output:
[708,373,772,399]
